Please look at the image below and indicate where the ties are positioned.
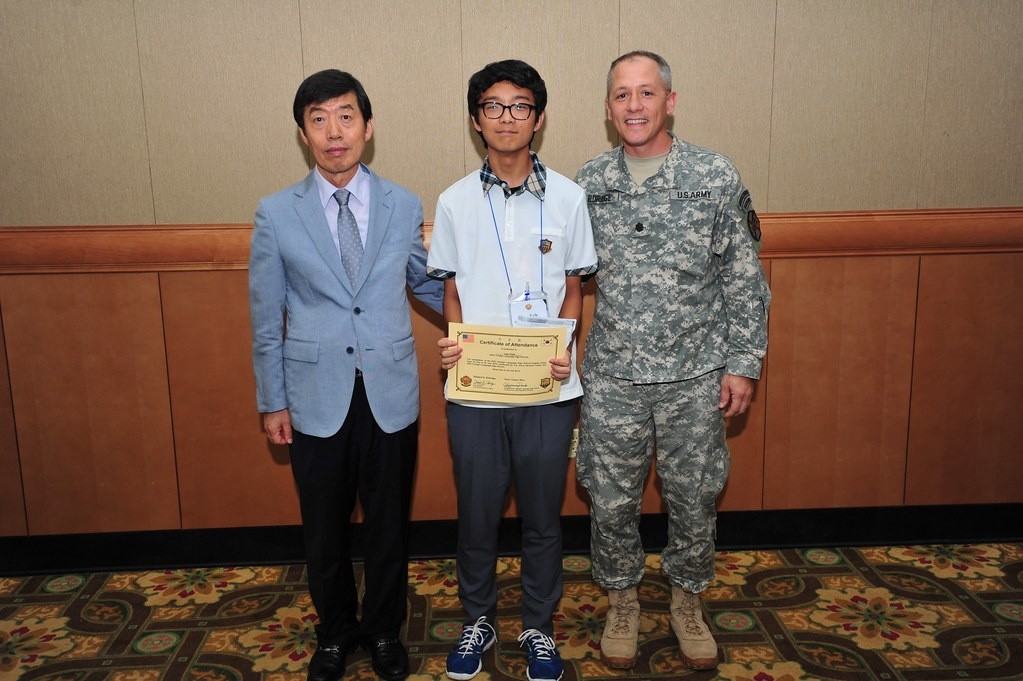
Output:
[332,189,363,371]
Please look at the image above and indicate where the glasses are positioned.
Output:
[478,101,537,120]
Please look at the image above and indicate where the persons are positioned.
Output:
[249,68,445,681]
[572,50,772,669]
[427,60,599,681]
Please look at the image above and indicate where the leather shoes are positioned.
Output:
[307,641,351,681]
[370,637,409,681]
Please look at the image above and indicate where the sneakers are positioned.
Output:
[600,587,641,668]
[669,587,719,669]
[446,616,498,680]
[518,629,564,681]
